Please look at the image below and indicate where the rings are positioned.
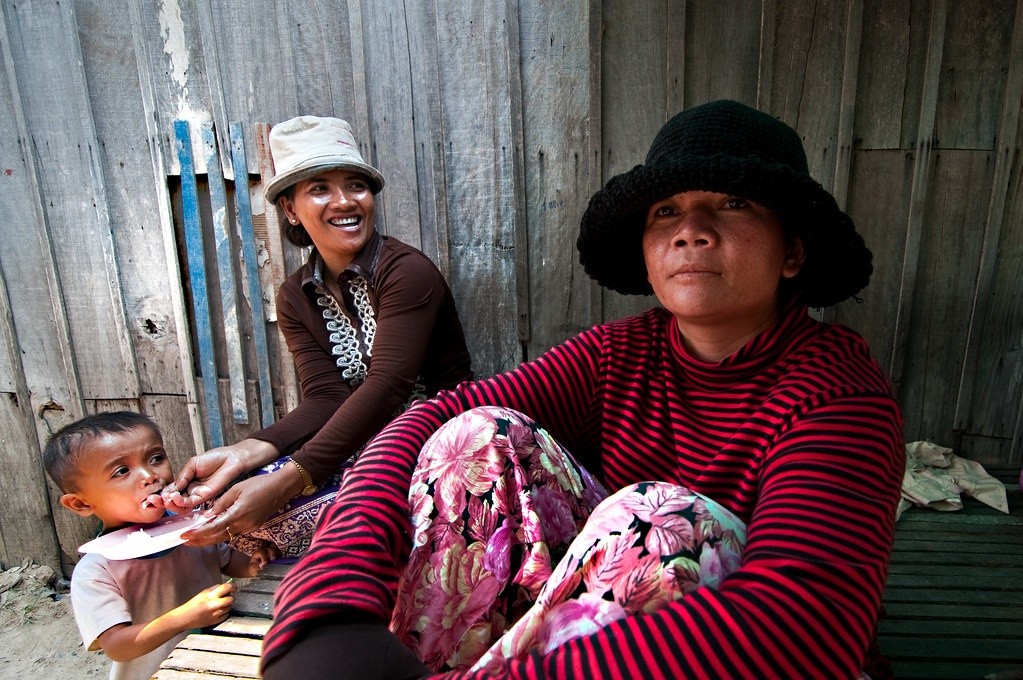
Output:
[226,527,235,541]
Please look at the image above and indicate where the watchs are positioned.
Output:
[289,458,317,496]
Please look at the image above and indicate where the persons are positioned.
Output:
[146,114,476,565]
[259,99,907,679]
[43,410,277,680]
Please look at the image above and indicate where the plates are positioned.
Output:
[77,507,216,560]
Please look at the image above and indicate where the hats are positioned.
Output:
[263,115,386,205]
[576,99,872,309]
